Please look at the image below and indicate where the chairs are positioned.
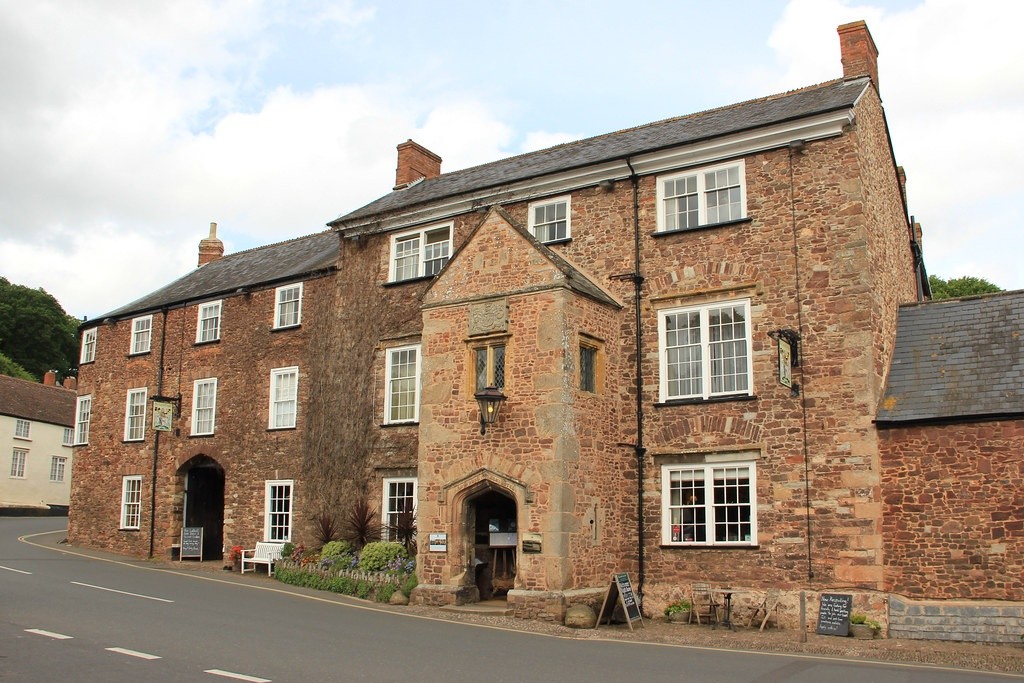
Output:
[688,582,721,627]
[746,588,783,633]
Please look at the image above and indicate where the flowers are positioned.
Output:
[229,545,252,562]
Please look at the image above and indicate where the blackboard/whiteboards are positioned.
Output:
[816,594,852,636]
[180,527,203,557]
[600,572,641,622]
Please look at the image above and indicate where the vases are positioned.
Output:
[231,561,242,573]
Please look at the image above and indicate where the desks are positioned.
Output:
[707,588,750,632]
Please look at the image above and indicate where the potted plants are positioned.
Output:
[849,613,882,640]
[664,599,692,626]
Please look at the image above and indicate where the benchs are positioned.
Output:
[240,541,286,577]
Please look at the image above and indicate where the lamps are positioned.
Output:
[103,318,117,328]
[790,139,805,149]
[600,179,615,186]
[236,288,250,295]
[473,383,509,437]
[478,206,490,214]
[351,235,360,242]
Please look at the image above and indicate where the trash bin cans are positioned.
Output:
[229,546,248,570]
